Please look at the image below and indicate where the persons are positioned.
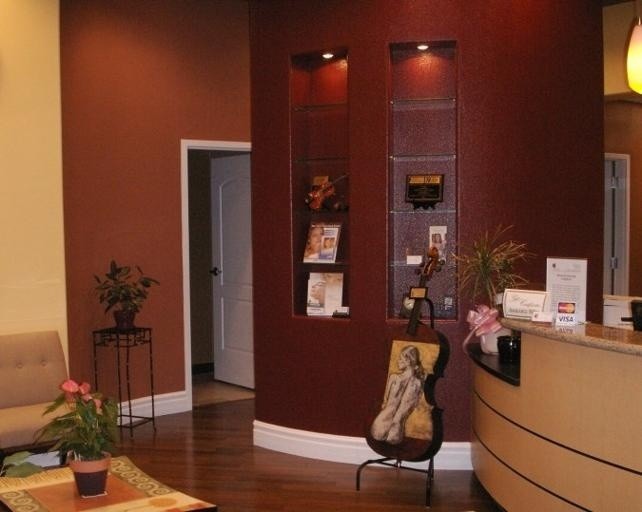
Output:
[370,343,428,447]
[310,281,325,307]
[322,238,334,253]
[304,227,324,258]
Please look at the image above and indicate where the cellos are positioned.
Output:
[364,246,450,463]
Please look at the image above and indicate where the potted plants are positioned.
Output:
[91,259,161,329]
[446,221,540,356]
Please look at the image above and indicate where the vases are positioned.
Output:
[66,449,111,497]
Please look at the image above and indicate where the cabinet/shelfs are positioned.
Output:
[391,42,458,322]
[290,50,349,318]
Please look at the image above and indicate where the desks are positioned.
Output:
[91,326,156,439]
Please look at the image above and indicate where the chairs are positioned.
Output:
[0,330,76,470]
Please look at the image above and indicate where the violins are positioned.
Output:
[305,172,349,211]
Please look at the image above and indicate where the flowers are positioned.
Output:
[30,378,122,460]
